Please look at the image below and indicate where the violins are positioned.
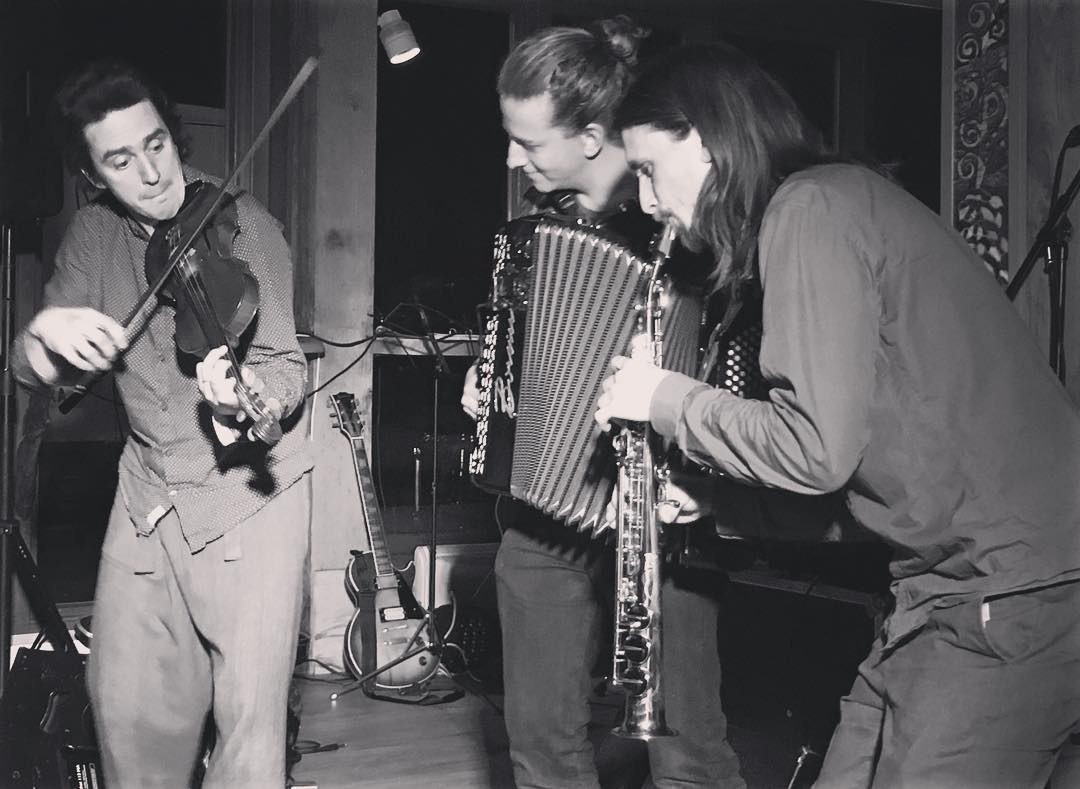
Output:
[145,178,283,445]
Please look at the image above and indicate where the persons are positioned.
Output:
[593,43,1079,789]
[461,11,747,789]
[8,56,315,789]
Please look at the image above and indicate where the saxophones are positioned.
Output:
[602,214,686,740]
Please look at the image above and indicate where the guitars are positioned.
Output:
[325,390,445,699]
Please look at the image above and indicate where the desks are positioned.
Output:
[372,330,483,642]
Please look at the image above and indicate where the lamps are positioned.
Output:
[377,10,420,64]
